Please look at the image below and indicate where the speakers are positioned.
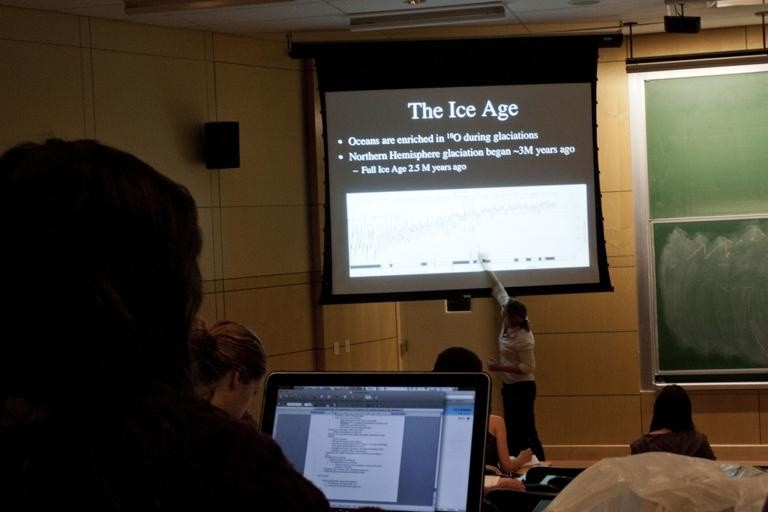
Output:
[664,16,700,33]
[204,121,240,169]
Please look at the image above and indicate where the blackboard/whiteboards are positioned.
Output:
[632,65,768,391]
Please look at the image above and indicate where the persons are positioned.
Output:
[431,346,526,499]
[484,413,535,478]
[485,270,545,461]
[1,136,330,511]
[629,384,718,460]
[183,319,267,437]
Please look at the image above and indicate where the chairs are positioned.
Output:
[483,467,587,511]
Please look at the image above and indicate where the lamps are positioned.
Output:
[350,6,508,32]
[664,1,701,32]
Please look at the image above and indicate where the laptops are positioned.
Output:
[258,370,492,512]
